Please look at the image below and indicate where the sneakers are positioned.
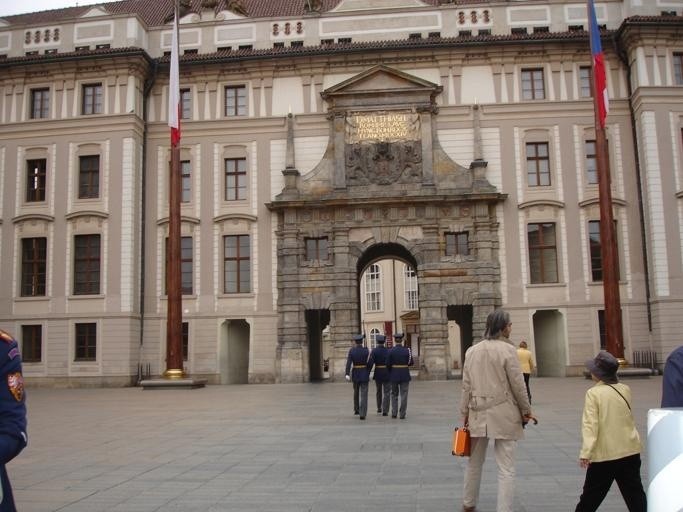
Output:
[463,504,476,511]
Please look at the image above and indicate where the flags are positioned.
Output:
[586,0,611,135]
[163,0,188,152]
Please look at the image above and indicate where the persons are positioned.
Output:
[659,343,681,409]
[385,331,414,420]
[345,334,371,421]
[575,351,648,511]
[0,330,28,511]
[517,339,536,407]
[344,110,357,145]
[366,332,392,417]
[408,107,420,142]
[457,308,532,511]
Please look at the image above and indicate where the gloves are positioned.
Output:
[346,374,350,381]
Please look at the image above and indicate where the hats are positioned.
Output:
[352,335,363,341]
[394,334,403,339]
[376,335,386,342]
[584,351,620,384]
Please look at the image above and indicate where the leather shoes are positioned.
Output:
[360,416,365,419]
[354,412,359,414]
[378,410,406,419]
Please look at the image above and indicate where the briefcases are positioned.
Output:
[452,426,470,457]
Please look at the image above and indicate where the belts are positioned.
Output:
[353,365,367,368]
[391,365,409,368]
[375,366,386,368]
